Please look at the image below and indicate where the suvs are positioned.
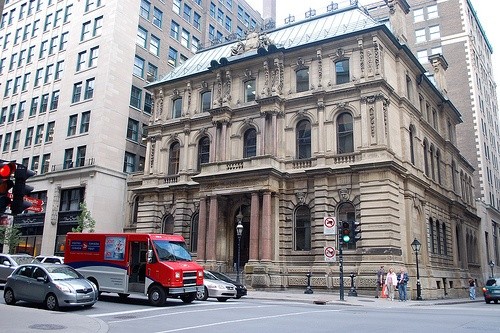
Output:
[482,278,500,304]
[0,253,42,287]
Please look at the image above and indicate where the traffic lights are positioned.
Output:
[351,220,362,243]
[341,221,350,243]
[0,159,17,215]
[10,165,36,216]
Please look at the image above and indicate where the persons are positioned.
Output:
[385,268,397,301]
[397,267,409,302]
[469,278,476,300]
[374,266,385,298]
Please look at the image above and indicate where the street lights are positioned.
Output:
[488,261,495,277]
[236,221,243,282]
[411,237,422,301]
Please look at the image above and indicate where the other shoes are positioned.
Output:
[387,298,394,301]
[399,299,405,301]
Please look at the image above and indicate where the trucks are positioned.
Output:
[63,231,204,305]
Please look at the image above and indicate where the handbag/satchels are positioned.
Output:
[384,285,389,295]
[399,279,406,284]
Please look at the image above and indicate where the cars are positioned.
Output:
[3,263,99,310]
[206,269,248,299]
[195,270,237,302]
[30,255,64,266]
